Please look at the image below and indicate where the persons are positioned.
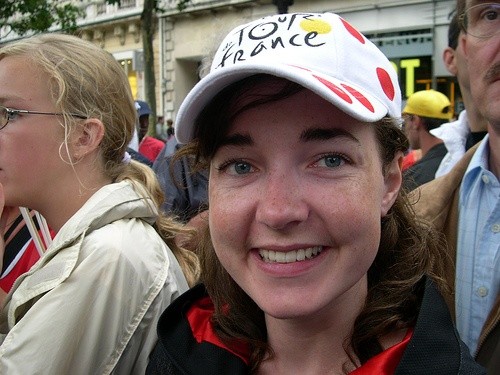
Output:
[399,0,500,375]
[125,13,488,224]
[0,34,199,375]
[1,206,56,307]
[144,12,485,375]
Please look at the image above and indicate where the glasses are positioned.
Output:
[0,106,88,130]
[457,3,500,38]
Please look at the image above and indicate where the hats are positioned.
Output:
[174,12,403,144]
[133,100,154,118]
[403,89,455,121]
